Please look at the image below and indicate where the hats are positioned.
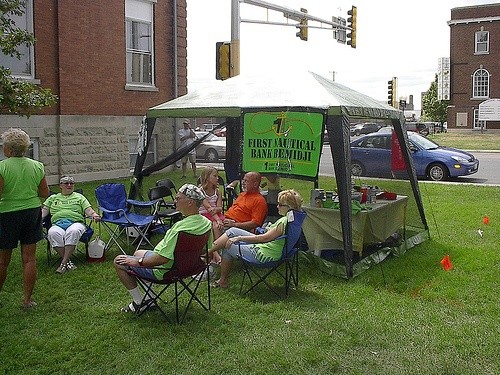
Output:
[60,177,75,183]
[183,119,190,124]
[179,184,206,201]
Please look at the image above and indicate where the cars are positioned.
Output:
[322,121,442,143]
[193,122,226,162]
[351,133,479,182]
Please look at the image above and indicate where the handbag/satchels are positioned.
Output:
[56,218,72,229]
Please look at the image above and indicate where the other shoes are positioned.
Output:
[194,176,199,178]
[66,261,76,270]
[182,176,186,179]
[192,269,216,282]
[56,262,66,274]
[24,302,37,308]
[210,256,222,266]
[212,281,228,288]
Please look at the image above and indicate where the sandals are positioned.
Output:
[143,298,157,311]
[120,299,148,313]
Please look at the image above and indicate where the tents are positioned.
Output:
[127,44,441,287]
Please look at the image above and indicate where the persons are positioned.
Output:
[192,172,268,281]
[196,166,225,266]
[42,176,101,273]
[178,119,200,178]
[200,190,302,288]
[0,128,50,309]
[113,184,213,312]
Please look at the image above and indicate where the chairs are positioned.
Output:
[148,186,184,235]
[156,179,185,227]
[42,188,94,270]
[95,182,159,256]
[115,229,211,327]
[232,208,307,301]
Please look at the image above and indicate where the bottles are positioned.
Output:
[314,196,322,206]
[351,178,377,207]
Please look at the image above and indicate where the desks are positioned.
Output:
[301,192,409,258]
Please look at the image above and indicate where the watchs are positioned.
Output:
[138,256,145,267]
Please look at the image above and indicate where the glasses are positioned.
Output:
[64,182,74,184]
[277,201,288,208]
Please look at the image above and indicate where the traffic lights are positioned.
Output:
[295,9,308,41]
[218,45,229,79]
[346,6,358,48]
[388,77,397,107]
